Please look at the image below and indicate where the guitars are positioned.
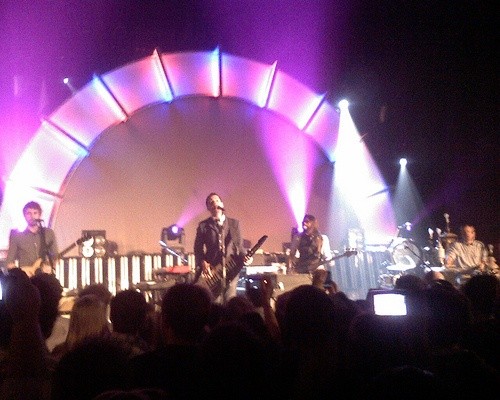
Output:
[294,247,358,274]
[20,233,92,279]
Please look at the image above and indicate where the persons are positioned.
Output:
[443,224,486,285]
[7,201,58,274]
[288,215,326,288]
[0,268,500,400]
[194,193,253,305]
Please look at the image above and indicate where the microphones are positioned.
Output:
[33,219,44,223]
[216,206,225,211]
[303,225,308,237]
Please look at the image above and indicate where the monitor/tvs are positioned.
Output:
[368,290,411,320]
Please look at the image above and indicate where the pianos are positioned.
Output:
[205,235,268,298]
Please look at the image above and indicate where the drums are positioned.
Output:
[392,240,423,265]
